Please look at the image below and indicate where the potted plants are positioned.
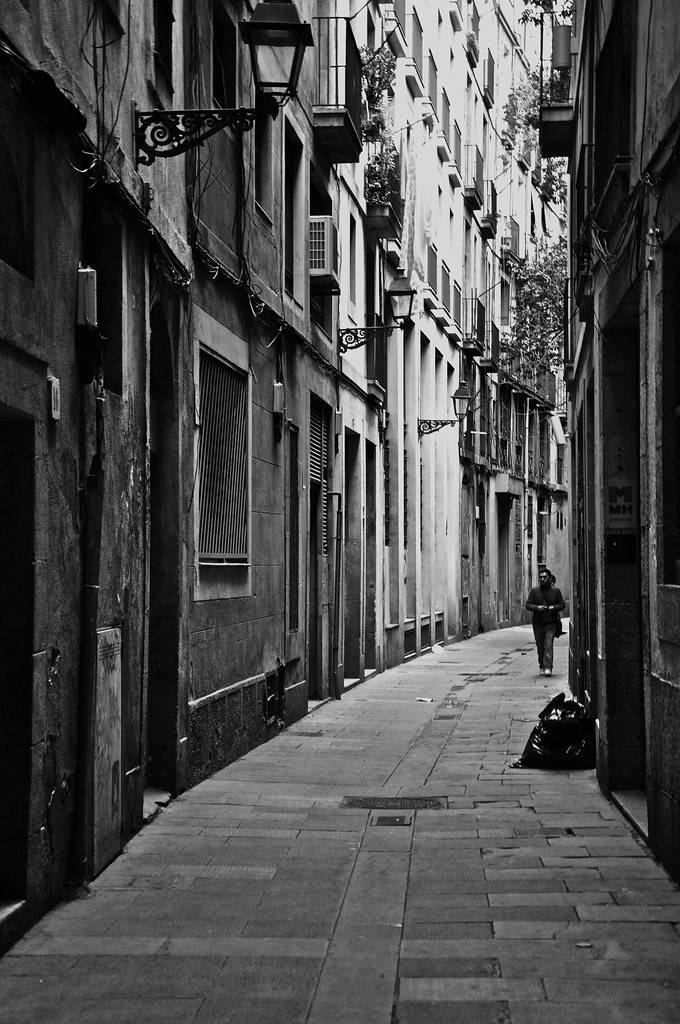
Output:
[358,44,398,134]
[363,149,403,239]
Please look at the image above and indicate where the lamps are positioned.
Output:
[130,0,315,172]
[337,266,418,359]
[552,24,579,71]
[550,415,570,445]
[417,380,473,442]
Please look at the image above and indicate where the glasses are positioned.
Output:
[539,575,549,579]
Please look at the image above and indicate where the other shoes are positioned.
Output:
[540,668,544,675]
[544,668,552,677]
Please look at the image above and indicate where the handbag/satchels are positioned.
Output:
[554,615,567,638]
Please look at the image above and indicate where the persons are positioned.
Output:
[526,569,566,676]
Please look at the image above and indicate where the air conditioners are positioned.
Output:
[309,215,342,290]
